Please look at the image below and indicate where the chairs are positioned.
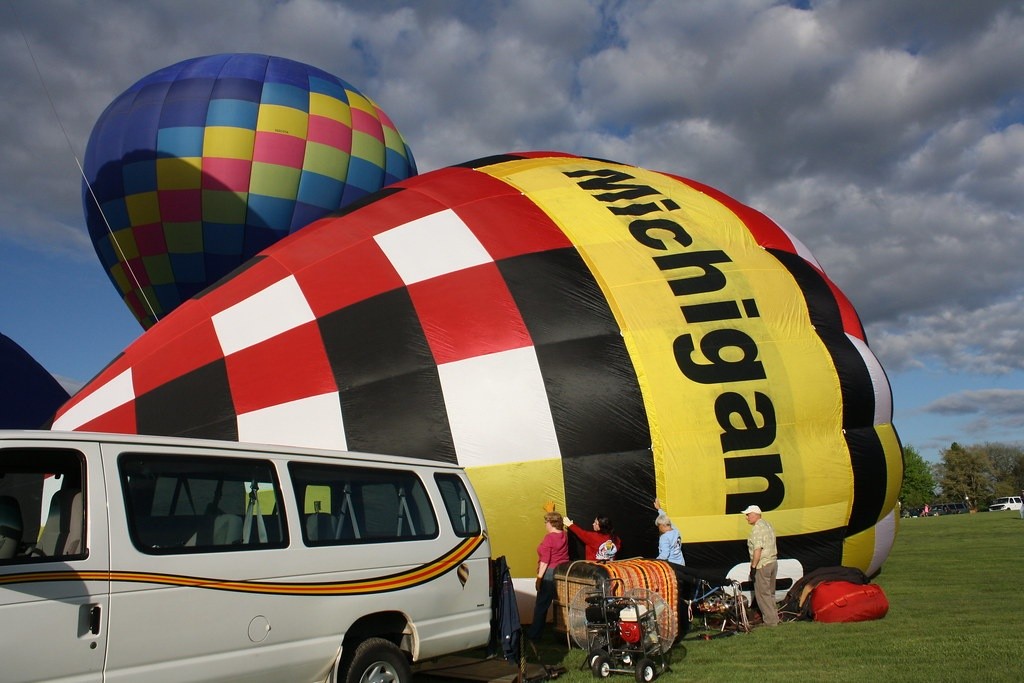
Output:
[31,485,83,557]
[0,495,24,560]
[185,514,243,546]
[306,513,338,540]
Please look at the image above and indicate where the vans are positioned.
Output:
[926,501,969,518]
[0,429,496,683]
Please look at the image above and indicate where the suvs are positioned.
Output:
[988,495,1023,514]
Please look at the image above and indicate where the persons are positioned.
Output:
[898,499,975,519]
[741,505,780,627]
[1019,490,1024,519]
[653,498,686,567]
[524,501,621,641]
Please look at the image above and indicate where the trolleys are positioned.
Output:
[588,579,670,679]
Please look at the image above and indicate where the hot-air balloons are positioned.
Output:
[77,51,419,331]
[1,152,903,625]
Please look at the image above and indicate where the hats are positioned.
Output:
[740,505,763,515]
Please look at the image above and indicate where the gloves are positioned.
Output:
[749,567,757,583]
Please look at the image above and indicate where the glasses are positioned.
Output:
[544,521,548,524]
